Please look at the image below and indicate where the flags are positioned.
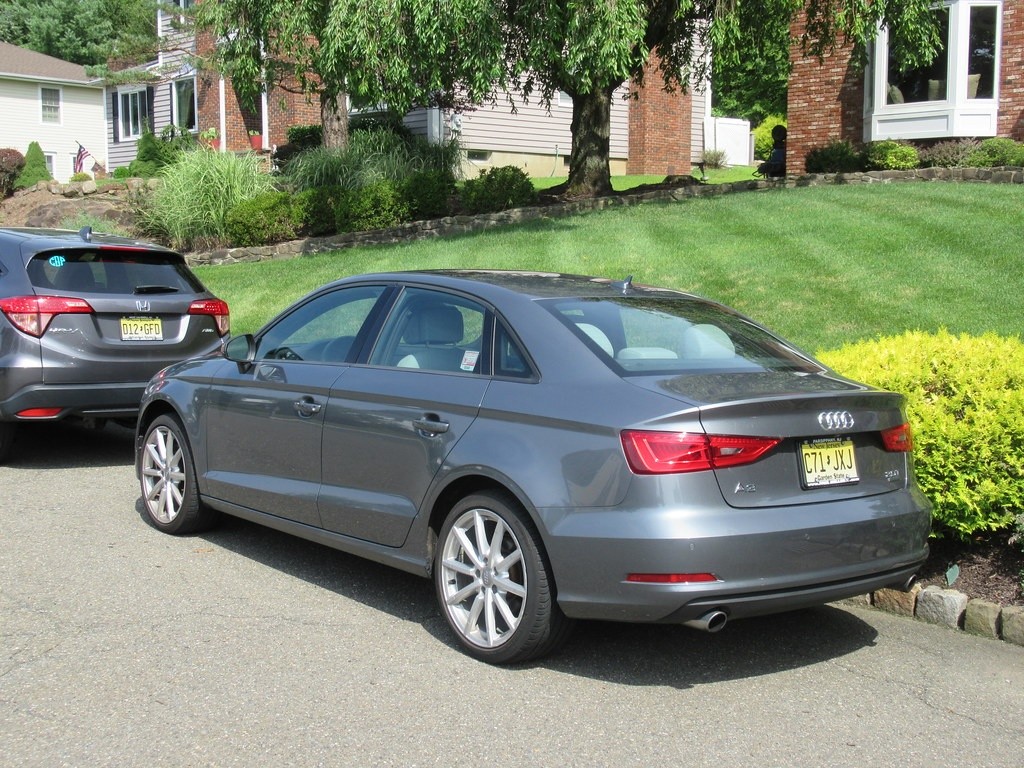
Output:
[76,145,91,173]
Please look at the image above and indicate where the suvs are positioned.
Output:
[0,222,231,450]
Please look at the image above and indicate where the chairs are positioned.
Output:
[682,318,736,361]
[574,323,615,359]
[396,300,466,367]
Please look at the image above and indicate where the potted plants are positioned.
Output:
[248,129,262,149]
[201,126,220,150]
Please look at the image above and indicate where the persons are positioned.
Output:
[758,141,786,177]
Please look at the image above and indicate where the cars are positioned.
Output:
[132,269,934,666]
